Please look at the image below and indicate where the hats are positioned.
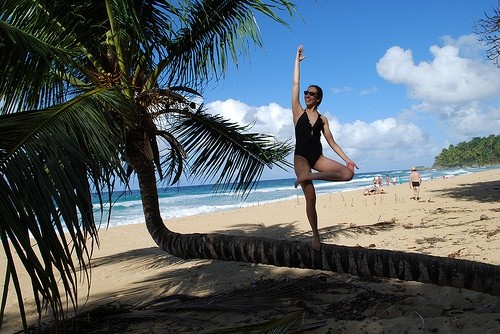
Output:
[411,167,416,171]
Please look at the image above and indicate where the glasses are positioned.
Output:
[304,91,317,96]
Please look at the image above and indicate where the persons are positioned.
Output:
[292,45,359,252]
[398,175,402,185]
[392,176,397,185]
[373,178,377,187]
[386,175,390,186]
[377,176,382,187]
[410,168,422,201]
